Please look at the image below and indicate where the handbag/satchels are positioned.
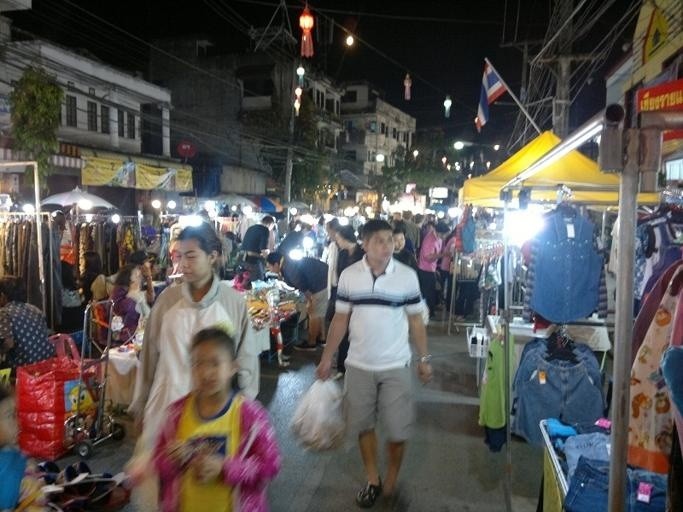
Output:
[16,334,101,461]
[435,271,442,290]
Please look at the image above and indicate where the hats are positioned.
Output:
[130,252,152,263]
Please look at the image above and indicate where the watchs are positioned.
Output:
[416,353,433,364]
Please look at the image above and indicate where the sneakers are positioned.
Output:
[316,337,326,348]
[293,340,317,352]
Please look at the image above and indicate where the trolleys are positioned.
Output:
[74,300,126,458]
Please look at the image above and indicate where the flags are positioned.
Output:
[476,64,507,132]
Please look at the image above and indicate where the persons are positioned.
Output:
[153,328,283,512]
[387,211,459,231]
[266,251,328,351]
[277,224,313,255]
[105,265,149,347]
[417,221,452,319]
[0,279,56,377]
[129,224,257,512]
[238,216,275,282]
[317,217,340,347]
[317,220,433,512]
[440,226,457,311]
[393,230,417,270]
[127,250,155,302]
[81,251,108,303]
[335,225,366,278]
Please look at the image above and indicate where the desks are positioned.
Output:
[251,286,307,356]
[100,321,270,433]
[485,314,607,448]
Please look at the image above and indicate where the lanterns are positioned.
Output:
[293,66,305,117]
[178,140,197,157]
[403,74,413,99]
[443,96,453,118]
[299,6,314,57]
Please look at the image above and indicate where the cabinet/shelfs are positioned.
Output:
[538,421,682,512]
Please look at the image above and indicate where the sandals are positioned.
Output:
[379,486,395,512]
[356,476,380,505]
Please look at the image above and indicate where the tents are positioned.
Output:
[447,131,660,335]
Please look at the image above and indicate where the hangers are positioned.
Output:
[482,244,502,260]
[546,325,579,364]
[555,184,578,220]
[463,204,474,223]
[72,214,133,224]
[1,212,47,221]
[659,185,683,222]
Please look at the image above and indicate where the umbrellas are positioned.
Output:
[41,186,118,224]
